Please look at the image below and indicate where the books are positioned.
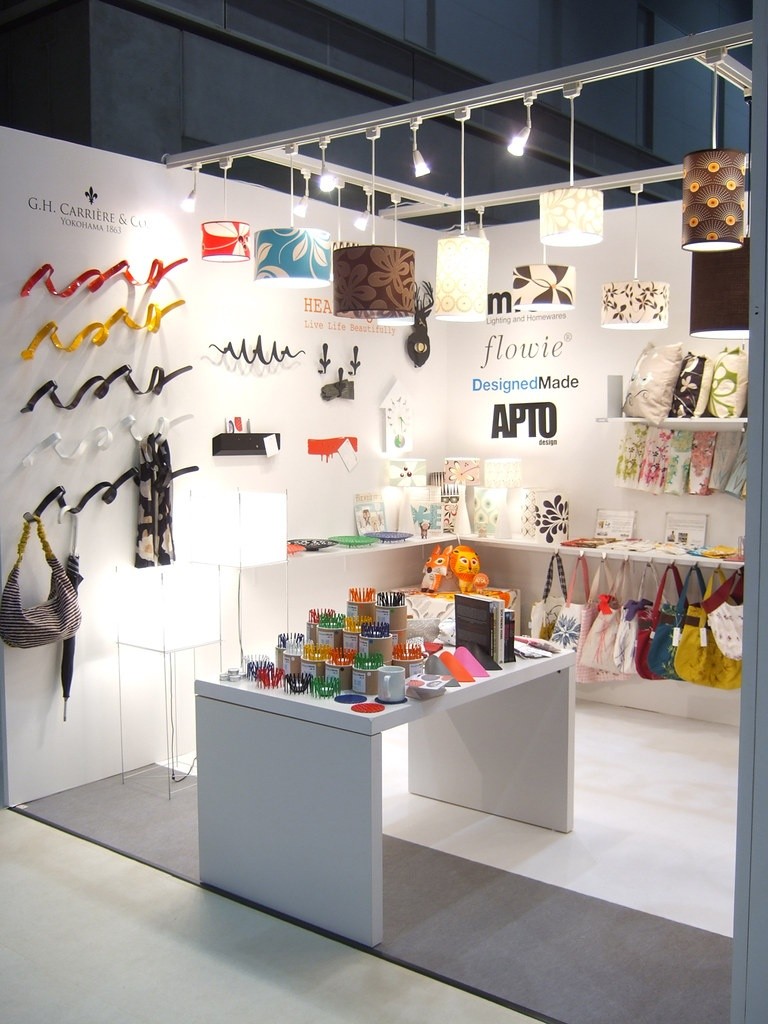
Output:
[454,594,516,664]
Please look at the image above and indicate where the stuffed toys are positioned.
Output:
[421,544,453,592]
[449,546,480,593]
[473,573,489,593]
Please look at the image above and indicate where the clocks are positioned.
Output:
[380,381,417,455]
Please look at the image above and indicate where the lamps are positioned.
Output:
[193,482,290,660]
[388,457,533,541]
[182,50,748,330]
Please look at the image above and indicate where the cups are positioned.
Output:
[377,667,405,702]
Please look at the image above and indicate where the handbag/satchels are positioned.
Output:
[529,553,744,688]
[0,514,81,649]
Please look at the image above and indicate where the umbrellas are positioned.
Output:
[58,505,84,721]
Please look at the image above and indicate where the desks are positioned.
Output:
[190,643,583,949]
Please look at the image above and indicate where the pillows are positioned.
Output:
[704,347,757,418]
[665,351,714,419]
[621,342,681,423]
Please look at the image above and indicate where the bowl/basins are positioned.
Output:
[425,642,443,654]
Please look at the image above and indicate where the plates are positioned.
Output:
[288,532,413,550]
